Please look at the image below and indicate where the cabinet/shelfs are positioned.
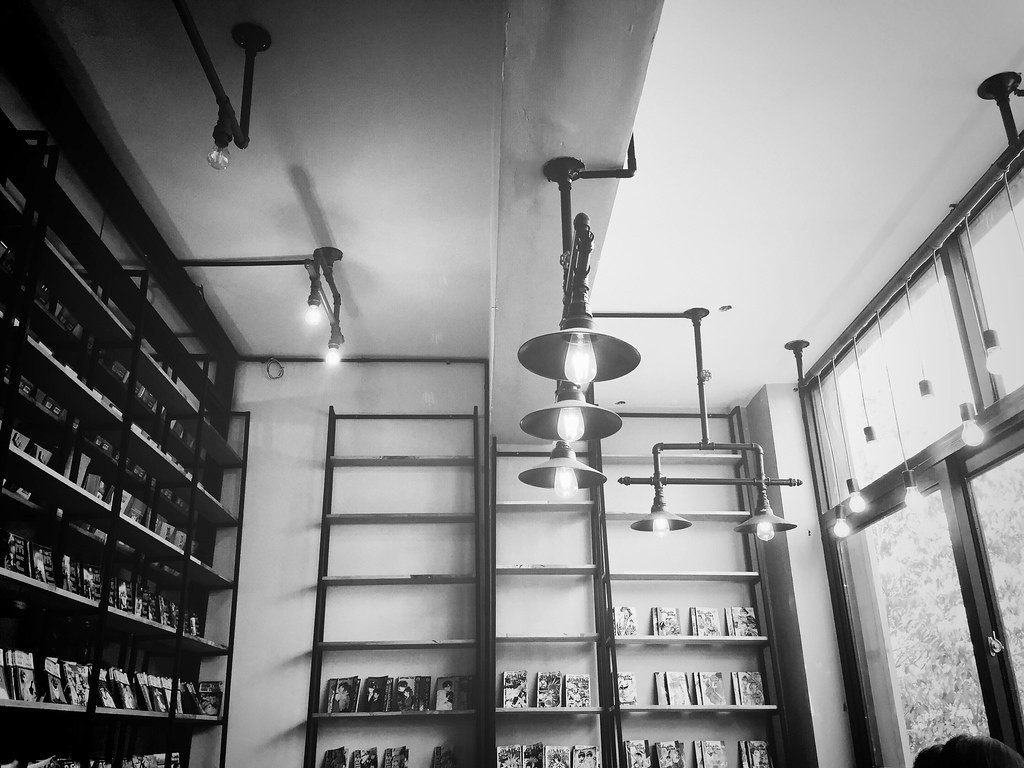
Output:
[300,405,793,768]
[1,108,252,768]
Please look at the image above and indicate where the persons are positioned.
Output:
[912,733,1024,767]
[0,430,224,768]
[323,604,769,768]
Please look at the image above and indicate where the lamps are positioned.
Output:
[515,158,806,543]
